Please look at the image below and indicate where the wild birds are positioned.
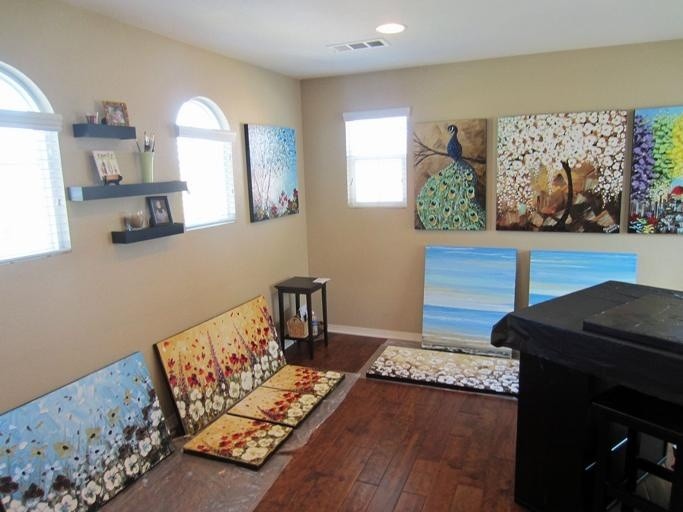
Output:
[416,125,487,231]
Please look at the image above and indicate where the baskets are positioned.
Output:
[286,315,310,339]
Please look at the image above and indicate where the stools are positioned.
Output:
[275,276,328,358]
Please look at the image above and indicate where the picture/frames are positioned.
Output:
[146,196,173,227]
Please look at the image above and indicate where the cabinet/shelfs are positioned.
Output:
[68,125,184,244]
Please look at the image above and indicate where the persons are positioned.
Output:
[154,200,170,223]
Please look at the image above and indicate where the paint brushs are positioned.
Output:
[136,131,155,153]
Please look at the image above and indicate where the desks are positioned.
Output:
[490,279,681,511]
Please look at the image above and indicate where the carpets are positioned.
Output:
[357,338,519,402]
[92,370,361,511]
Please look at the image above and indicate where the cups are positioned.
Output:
[86,112,98,124]
